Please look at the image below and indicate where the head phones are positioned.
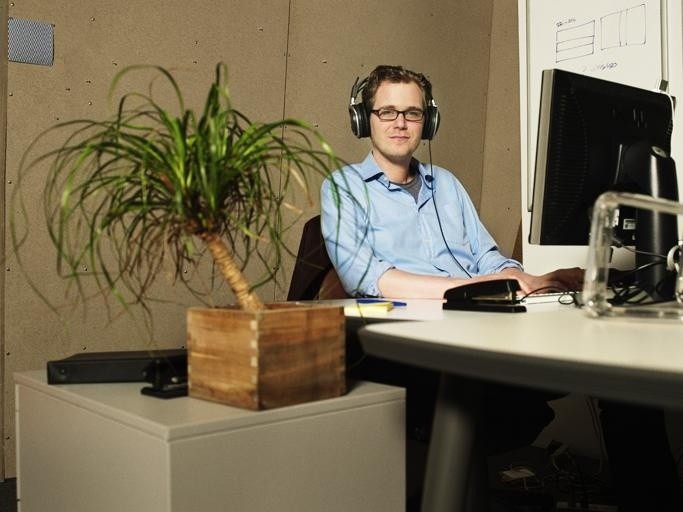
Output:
[348,74,439,139]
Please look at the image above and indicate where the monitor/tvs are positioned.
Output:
[529,68,678,302]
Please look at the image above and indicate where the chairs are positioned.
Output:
[288,214,555,511]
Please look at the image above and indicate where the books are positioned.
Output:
[343,301,394,313]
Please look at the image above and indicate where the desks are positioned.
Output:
[357,295,682,511]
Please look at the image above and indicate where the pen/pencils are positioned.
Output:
[357,299,408,306]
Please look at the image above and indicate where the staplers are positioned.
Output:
[442,279,527,313]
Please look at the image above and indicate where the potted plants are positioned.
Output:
[0,56,378,413]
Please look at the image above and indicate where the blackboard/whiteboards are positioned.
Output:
[517,0,683,279]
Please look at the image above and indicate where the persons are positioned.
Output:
[319,61,587,510]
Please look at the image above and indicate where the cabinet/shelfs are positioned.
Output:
[12,369,411,511]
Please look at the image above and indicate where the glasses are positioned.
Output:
[365,108,425,122]
